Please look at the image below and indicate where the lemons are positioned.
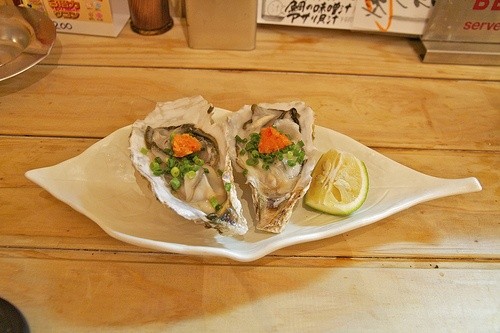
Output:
[303,147,369,218]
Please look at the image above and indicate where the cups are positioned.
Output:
[128,0,174,36]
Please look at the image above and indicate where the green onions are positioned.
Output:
[139,126,305,210]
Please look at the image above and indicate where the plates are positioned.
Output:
[24,105,483,262]
[0,5,57,82]
